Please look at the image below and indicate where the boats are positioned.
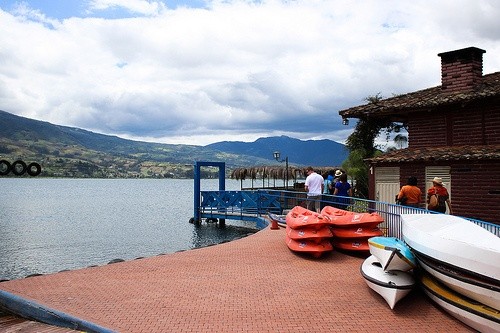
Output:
[284,205,500,333]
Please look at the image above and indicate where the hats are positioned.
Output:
[431,177,443,185]
[334,169,343,176]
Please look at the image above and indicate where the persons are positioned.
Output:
[426,175,455,216]
[331,169,342,194]
[304,167,324,213]
[332,173,353,210]
[321,174,334,195]
[398,176,421,209]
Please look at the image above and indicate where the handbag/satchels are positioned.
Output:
[395,195,407,206]
[428,186,439,210]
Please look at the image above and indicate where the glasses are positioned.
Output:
[305,171,309,173]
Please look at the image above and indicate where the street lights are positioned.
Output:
[273,148,289,190]
